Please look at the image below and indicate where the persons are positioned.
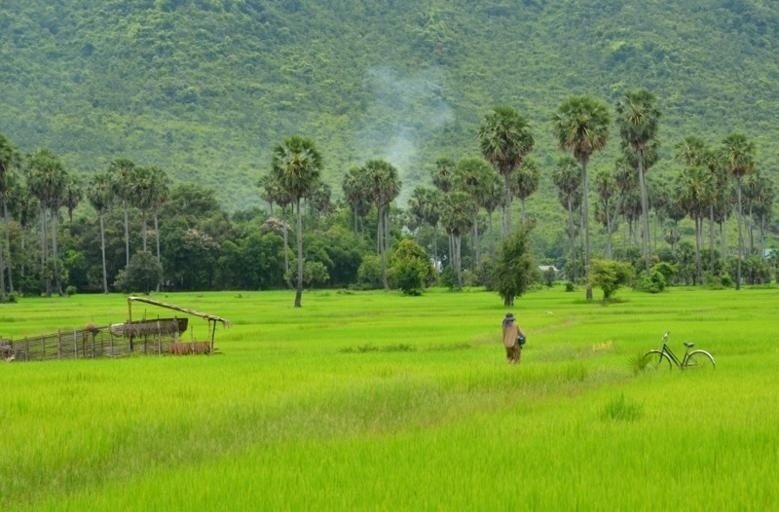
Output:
[502,313,526,364]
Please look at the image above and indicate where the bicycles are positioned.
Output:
[636,323,720,383]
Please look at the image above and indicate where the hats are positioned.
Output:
[506,313,515,321]
